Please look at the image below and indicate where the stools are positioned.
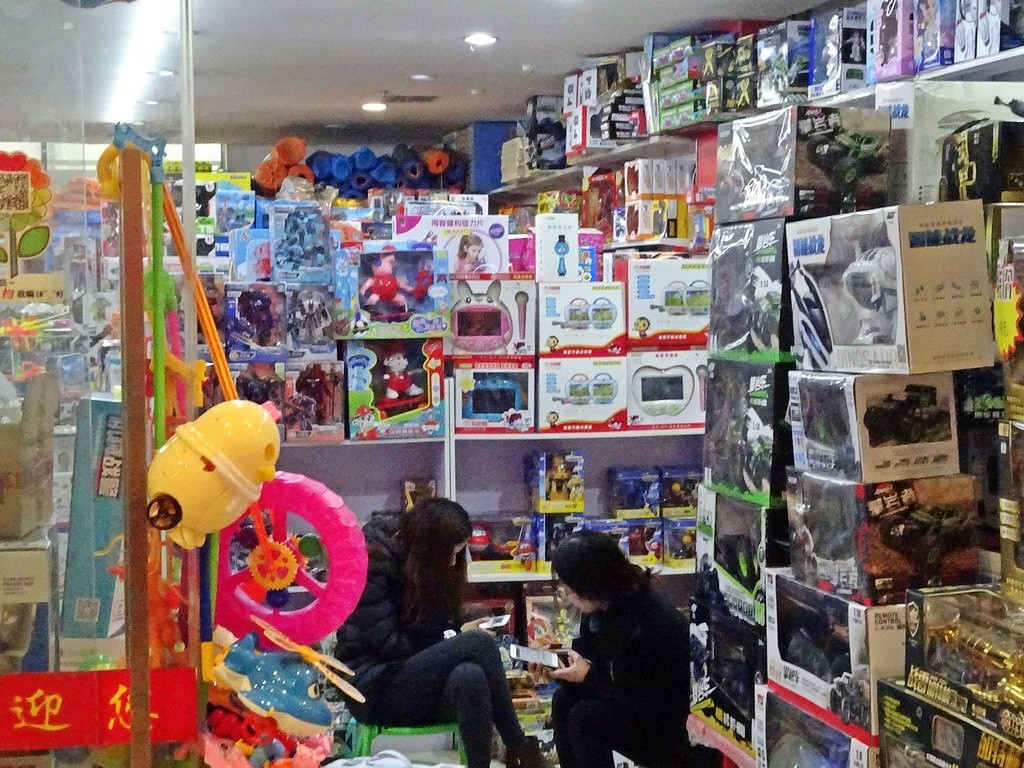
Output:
[354,723,469,766]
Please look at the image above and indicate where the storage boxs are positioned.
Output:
[0,0,1024,768]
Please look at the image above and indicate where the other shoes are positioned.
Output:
[505,735,551,768]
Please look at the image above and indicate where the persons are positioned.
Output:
[456,234,488,275]
[332,493,554,768]
[527,528,726,768]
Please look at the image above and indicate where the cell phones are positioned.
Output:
[509,644,558,667]
[479,615,511,629]
